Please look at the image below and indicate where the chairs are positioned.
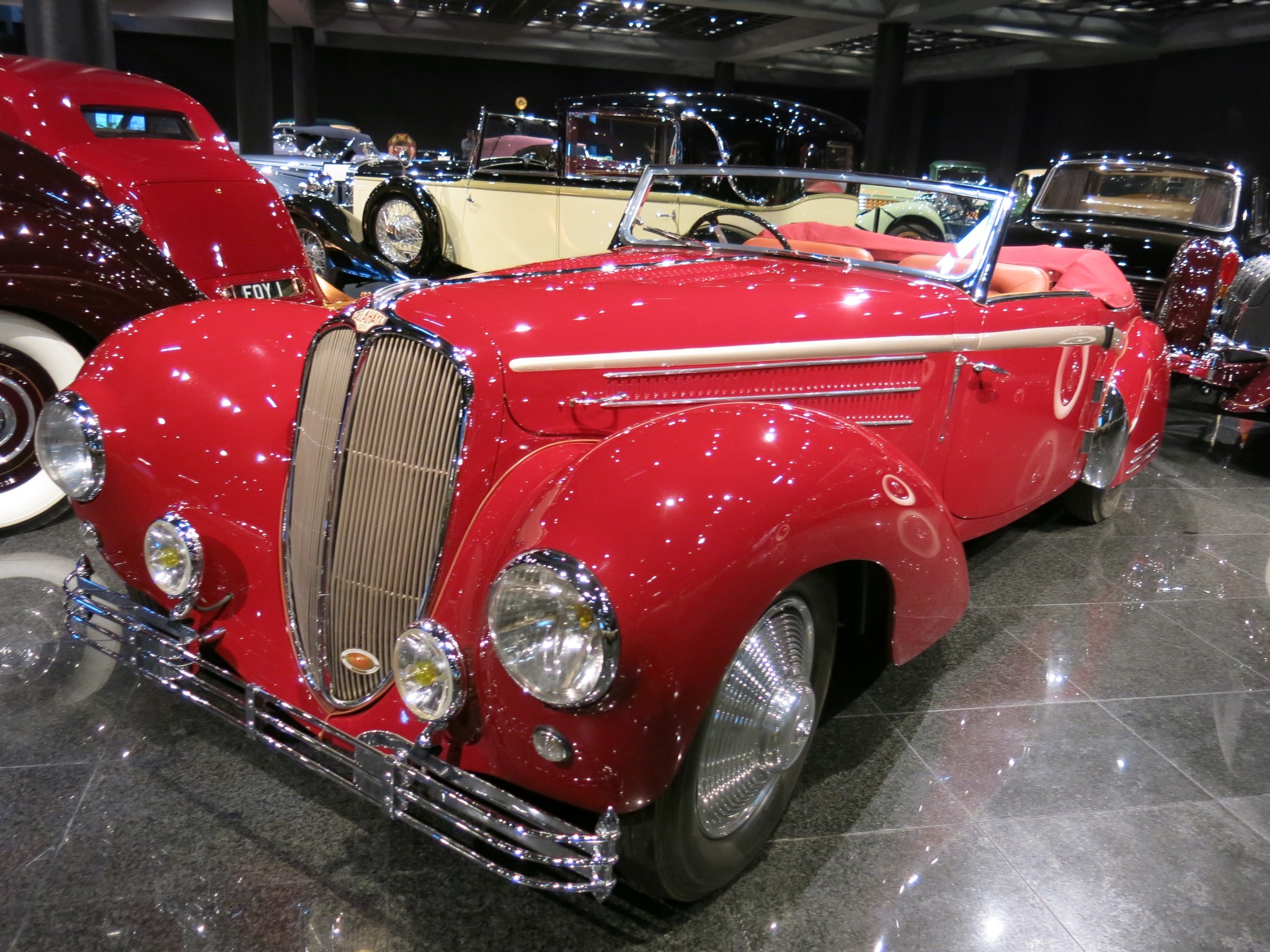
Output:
[896,254,1051,300]
[742,234,875,266]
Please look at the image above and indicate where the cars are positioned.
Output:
[1014,151,1270,458]
[30,163,1170,921]
[0,53,324,536]
[241,84,995,292]
